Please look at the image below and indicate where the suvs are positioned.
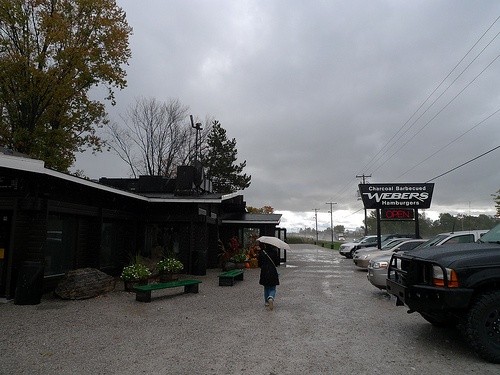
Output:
[385,222,500,364]
[339,235,382,258]
[356,233,423,250]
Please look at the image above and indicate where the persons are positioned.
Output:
[258,242,280,308]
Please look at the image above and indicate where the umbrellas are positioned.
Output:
[256,236,292,251]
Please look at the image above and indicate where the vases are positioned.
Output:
[159,273,180,282]
[123,277,149,292]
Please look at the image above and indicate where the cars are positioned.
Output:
[353,239,429,268]
[367,217,490,291]
[354,239,394,258]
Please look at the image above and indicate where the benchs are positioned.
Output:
[217,268,245,287]
[133,278,202,303]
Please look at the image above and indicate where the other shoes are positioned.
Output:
[265,301,269,306]
[268,297,274,310]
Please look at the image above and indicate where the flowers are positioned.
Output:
[119,261,152,280]
[156,256,183,273]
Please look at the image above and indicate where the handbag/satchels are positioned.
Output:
[278,272,280,280]
